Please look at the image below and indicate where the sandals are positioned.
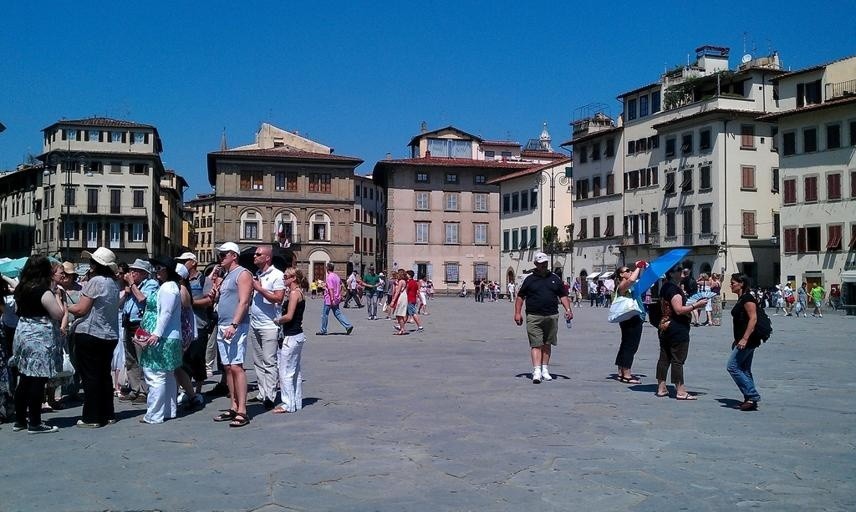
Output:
[213,408,236,421]
[622,376,643,384]
[229,413,250,427]
[617,375,622,381]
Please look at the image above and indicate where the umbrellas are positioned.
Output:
[630,249,692,301]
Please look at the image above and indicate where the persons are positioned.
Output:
[514,252,573,384]
[0,241,307,433]
[589,279,614,307]
[462,278,518,304]
[615,260,642,384]
[656,262,709,400]
[681,269,721,327]
[729,272,761,410]
[562,277,583,309]
[309,262,432,335]
[750,281,840,318]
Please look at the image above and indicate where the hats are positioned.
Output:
[81,246,118,271]
[174,263,189,279]
[174,252,197,262]
[534,252,548,263]
[776,284,784,290]
[128,258,152,275]
[215,242,240,256]
[148,255,175,269]
[63,261,80,277]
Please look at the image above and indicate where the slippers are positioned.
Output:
[677,393,697,400]
[657,390,674,397]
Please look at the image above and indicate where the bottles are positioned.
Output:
[275,303,281,318]
[566,309,572,328]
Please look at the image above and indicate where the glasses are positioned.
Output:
[154,266,167,271]
[218,252,226,257]
[253,252,262,256]
[623,268,631,273]
[283,274,292,279]
[677,265,682,272]
[115,271,124,276]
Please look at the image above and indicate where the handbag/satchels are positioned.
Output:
[131,326,162,351]
[647,300,663,329]
[608,294,643,323]
[122,312,131,327]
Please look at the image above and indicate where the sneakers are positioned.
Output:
[194,394,203,403]
[373,315,378,320]
[76,419,100,428]
[316,331,327,335]
[741,398,760,410]
[392,325,399,331]
[347,326,353,334]
[368,316,373,320]
[534,371,542,383]
[415,327,423,333]
[13,420,28,432]
[177,392,188,402]
[132,394,148,404]
[541,369,553,380]
[27,422,58,434]
[263,398,273,406]
[274,406,290,412]
[247,396,262,404]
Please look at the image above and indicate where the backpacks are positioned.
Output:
[755,302,774,343]
